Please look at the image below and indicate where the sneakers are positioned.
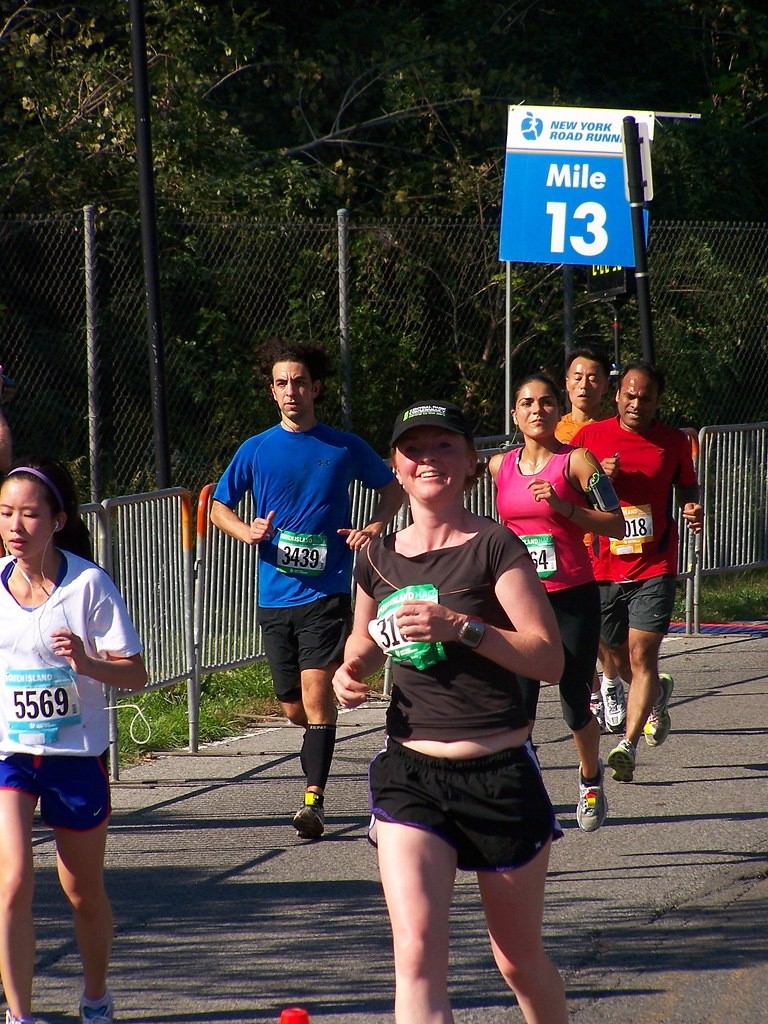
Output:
[5,1009,34,1024]
[644,673,674,747]
[602,682,627,733]
[608,741,636,783]
[79,995,113,1024]
[292,791,325,840]
[589,700,604,732]
[576,757,608,833]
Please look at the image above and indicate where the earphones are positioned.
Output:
[54,521,60,530]
[515,414,518,420]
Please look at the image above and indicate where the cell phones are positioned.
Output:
[592,474,618,510]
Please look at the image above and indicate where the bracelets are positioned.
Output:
[564,502,574,518]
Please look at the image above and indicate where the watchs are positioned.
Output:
[457,613,485,651]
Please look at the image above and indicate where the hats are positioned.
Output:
[388,401,467,447]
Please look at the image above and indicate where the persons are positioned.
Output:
[552,349,629,734]
[0,454,150,1024]
[567,359,705,782]
[210,348,405,839]
[332,400,572,1023]
[489,373,627,831]
